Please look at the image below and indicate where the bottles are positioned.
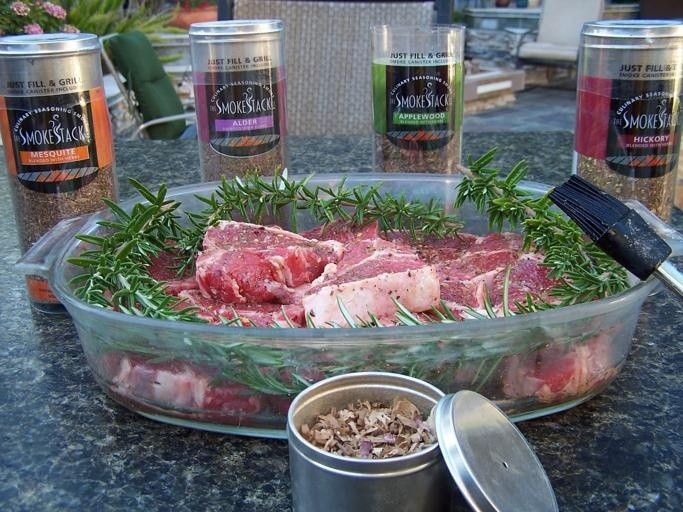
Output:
[188,18,289,179]
[368,22,467,174]
[570,19,682,224]
[0,32,119,313]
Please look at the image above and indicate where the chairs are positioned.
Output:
[499,0,602,92]
[96,28,197,138]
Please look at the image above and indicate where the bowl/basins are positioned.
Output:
[15,170,683,440]
